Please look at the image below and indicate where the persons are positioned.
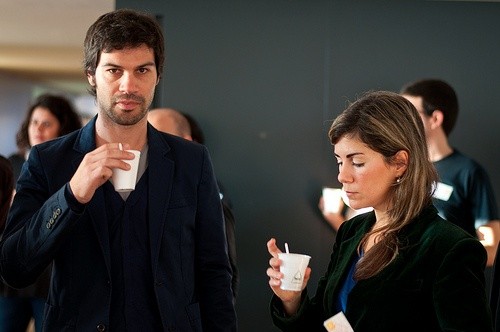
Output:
[319,79,500,266]
[0,9,235,332]
[0,94,239,332]
[267,90,493,332]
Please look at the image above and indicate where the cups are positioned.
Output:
[322,188,341,213]
[106,148,142,193]
[277,252,311,292]
